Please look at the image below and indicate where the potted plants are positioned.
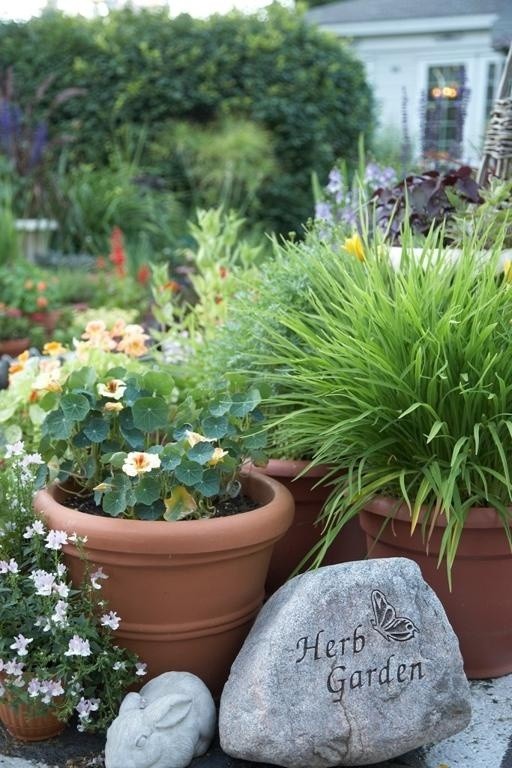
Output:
[222,179,511,680]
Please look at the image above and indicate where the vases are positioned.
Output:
[1,666,79,747]
[18,466,298,684]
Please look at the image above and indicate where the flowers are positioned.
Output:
[0,265,268,515]
[1,440,147,740]
